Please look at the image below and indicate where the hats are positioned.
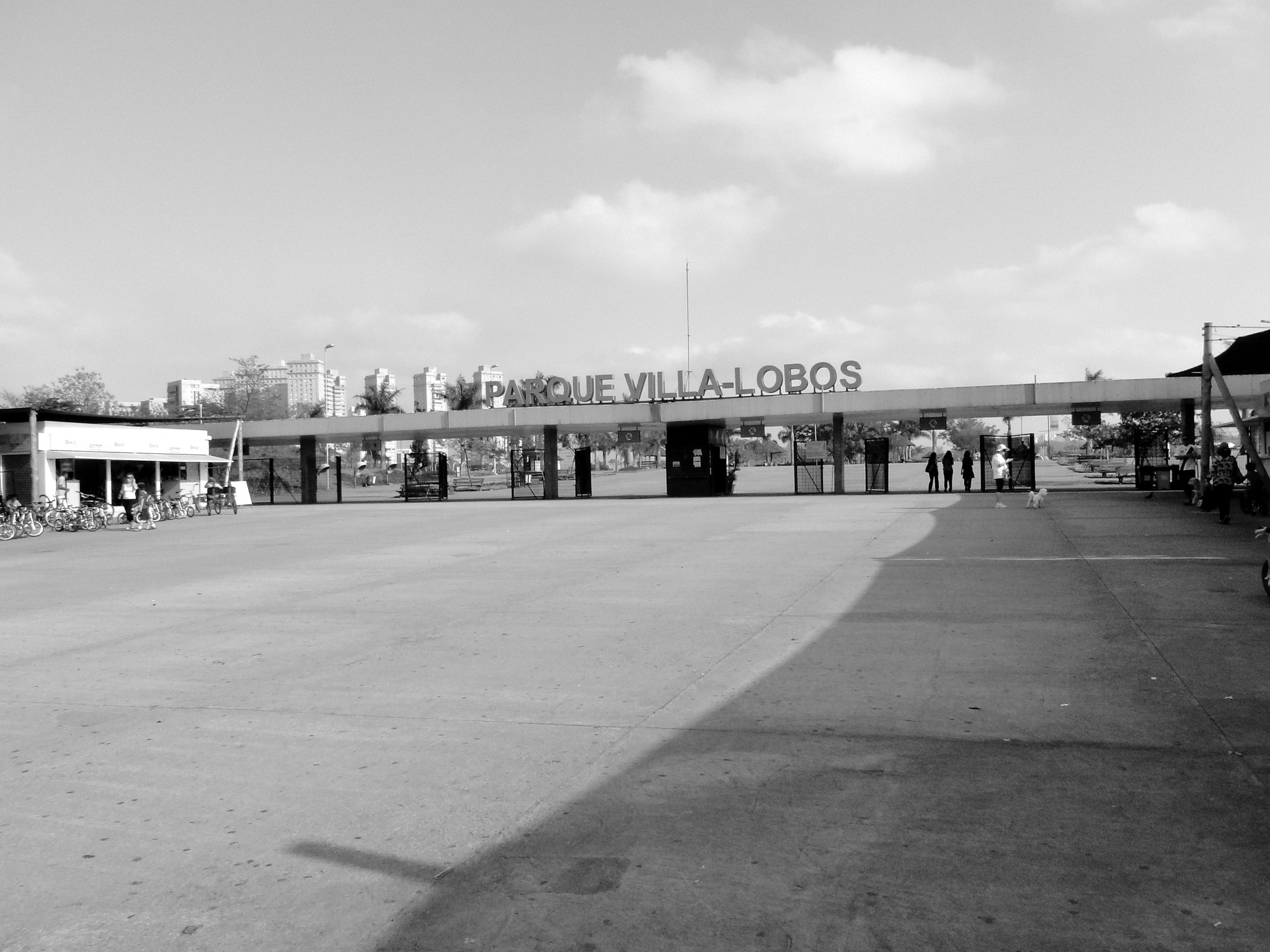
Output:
[1217,442,1231,454]
[996,444,1010,452]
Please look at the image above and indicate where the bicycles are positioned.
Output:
[0,478,238,541]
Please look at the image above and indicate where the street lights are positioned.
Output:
[324,344,335,490]
[491,364,499,474]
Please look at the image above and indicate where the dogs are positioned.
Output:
[1026,488,1048,509]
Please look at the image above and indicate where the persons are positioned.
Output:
[6,496,22,518]
[1173,437,1201,505]
[1208,444,1243,524]
[1243,462,1262,497]
[926,452,941,493]
[205,478,223,508]
[55,471,70,515]
[135,483,157,530]
[990,444,1014,508]
[118,474,138,524]
[961,451,974,491]
[942,451,954,492]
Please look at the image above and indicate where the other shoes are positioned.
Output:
[994,502,1007,508]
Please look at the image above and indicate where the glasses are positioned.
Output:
[1003,449,1006,452]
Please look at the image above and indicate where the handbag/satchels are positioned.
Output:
[1232,455,1244,484]
[145,494,154,506]
